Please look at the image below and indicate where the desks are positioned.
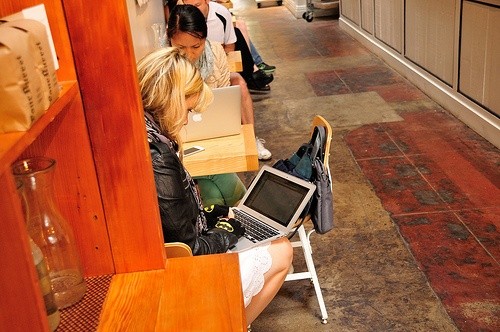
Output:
[176,124,259,177]
[225,50,242,72]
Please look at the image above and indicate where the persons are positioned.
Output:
[167,4,248,207]
[135,46,294,332]
[167,0,276,161]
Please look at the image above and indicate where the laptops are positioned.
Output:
[178,84,242,143]
[226,164,316,253]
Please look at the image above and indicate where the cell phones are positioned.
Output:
[183,146,206,157]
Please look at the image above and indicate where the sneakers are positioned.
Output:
[256,136,272,160]
[257,62,275,71]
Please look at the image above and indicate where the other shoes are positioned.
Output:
[250,69,274,91]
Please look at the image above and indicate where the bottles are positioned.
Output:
[9,157,87,332]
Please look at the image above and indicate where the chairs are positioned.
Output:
[229,114,333,323]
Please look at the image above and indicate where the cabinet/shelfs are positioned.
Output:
[0,0,248,332]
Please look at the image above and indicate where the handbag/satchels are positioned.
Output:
[312,126,333,234]
[272,143,316,218]
